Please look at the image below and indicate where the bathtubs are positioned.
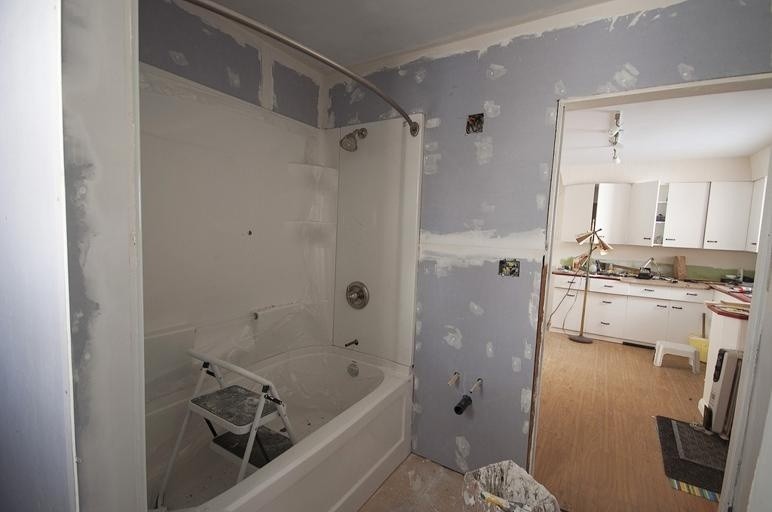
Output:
[145,347,415,512]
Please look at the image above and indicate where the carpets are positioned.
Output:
[655,413,729,504]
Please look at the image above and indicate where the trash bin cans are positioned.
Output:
[466,460,554,511]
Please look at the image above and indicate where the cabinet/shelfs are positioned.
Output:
[583,274,715,362]
[745,177,772,253]
[548,274,587,336]
[559,176,711,249]
[703,180,754,252]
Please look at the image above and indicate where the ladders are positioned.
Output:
[156,349,297,508]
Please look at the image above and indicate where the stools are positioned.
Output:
[653,340,700,374]
